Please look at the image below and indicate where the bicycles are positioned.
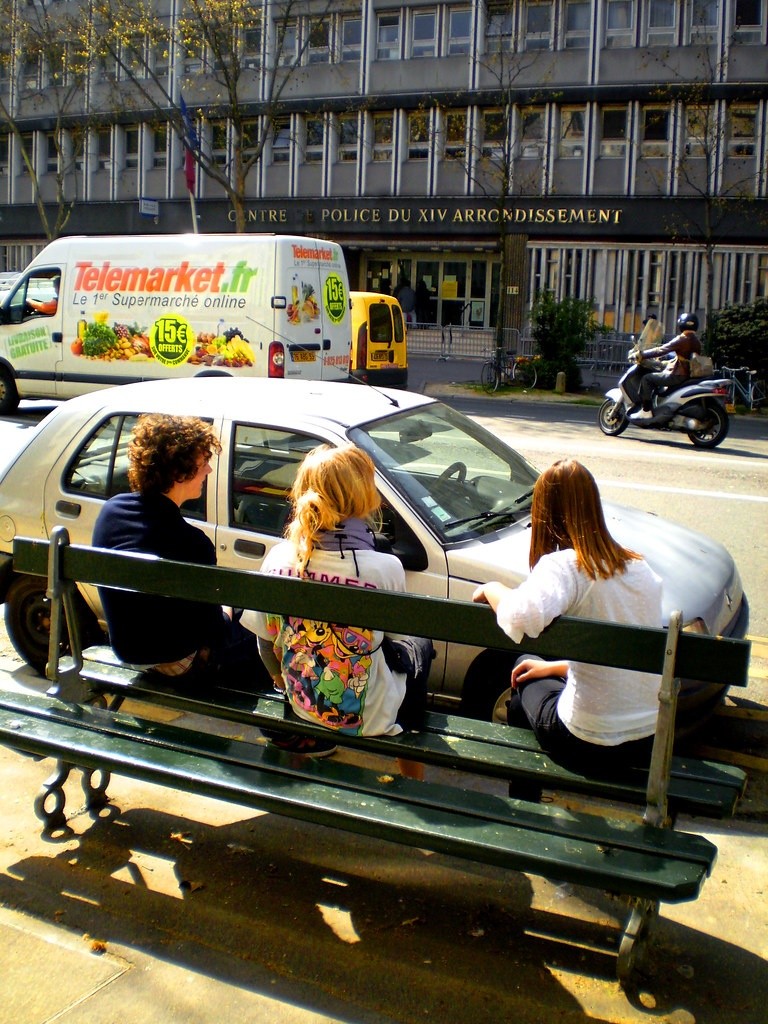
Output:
[720,365,768,415]
[479,346,538,394]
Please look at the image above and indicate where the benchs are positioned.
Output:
[0,526,753,990]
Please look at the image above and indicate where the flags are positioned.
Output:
[179,90,201,200]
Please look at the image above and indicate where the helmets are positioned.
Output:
[677,313,699,332]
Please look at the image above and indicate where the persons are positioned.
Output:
[239,443,435,784]
[25,276,60,316]
[91,413,338,760]
[628,312,701,418]
[472,457,705,831]
[392,277,431,334]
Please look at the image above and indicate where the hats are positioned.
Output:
[643,314,657,325]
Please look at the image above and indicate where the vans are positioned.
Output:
[0,232,352,419]
[346,291,408,391]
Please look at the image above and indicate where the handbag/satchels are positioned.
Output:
[678,352,712,377]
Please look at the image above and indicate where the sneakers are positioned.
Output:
[268,735,337,757]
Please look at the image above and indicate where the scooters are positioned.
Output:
[598,335,734,448]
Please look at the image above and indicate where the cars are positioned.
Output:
[0,379,753,720]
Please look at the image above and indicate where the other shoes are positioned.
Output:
[631,408,652,419]
